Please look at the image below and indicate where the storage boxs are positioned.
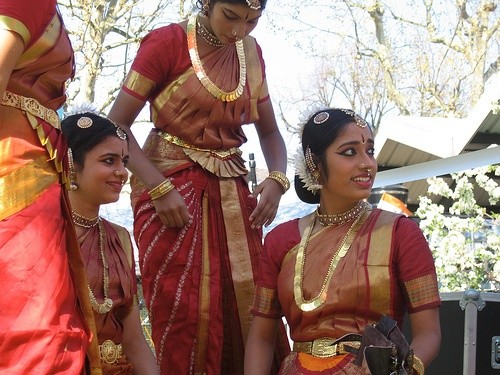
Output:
[404,291,500,375]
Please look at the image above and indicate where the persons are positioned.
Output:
[108,0,290,375]
[244,108,442,375]
[60,111,162,375]
[0,0,99,375]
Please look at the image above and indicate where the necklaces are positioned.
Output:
[71,211,113,314]
[294,201,371,312]
[187,14,246,102]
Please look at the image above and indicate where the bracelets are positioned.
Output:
[413,355,424,375]
[148,179,174,200]
[266,171,290,194]
